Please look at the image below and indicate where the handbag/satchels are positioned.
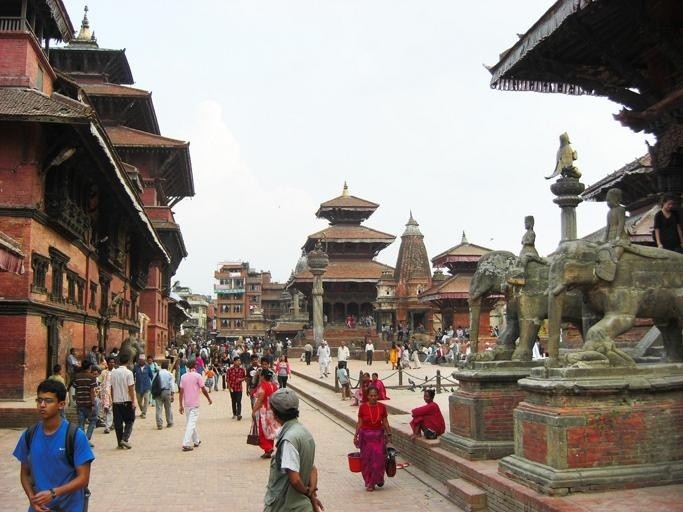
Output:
[424,428,437,439]
[247,434,260,445]
[386,447,396,477]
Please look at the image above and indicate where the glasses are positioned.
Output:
[35,397,59,404]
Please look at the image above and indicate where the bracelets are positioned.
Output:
[48,488,56,499]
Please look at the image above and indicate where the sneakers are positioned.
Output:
[117,440,132,449]
[140,412,146,418]
[260,448,274,459]
[232,415,242,420]
[104,425,115,433]
[88,439,94,446]
[158,423,173,429]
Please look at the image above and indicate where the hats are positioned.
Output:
[270,388,299,414]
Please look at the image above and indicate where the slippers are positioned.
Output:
[396,462,410,469]
[183,441,201,451]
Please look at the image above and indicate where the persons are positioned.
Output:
[97,337,230,448]
[338,340,349,361]
[352,386,394,493]
[600,188,627,243]
[652,196,683,254]
[347,315,424,369]
[225,336,291,420]
[427,323,500,366]
[337,363,390,406]
[263,386,323,512]
[532,337,549,361]
[13,378,94,512]
[317,340,330,378]
[304,342,313,365]
[49,344,105,443]
[519,215,540,257]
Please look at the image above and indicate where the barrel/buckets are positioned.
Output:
[348,453,361,472]
